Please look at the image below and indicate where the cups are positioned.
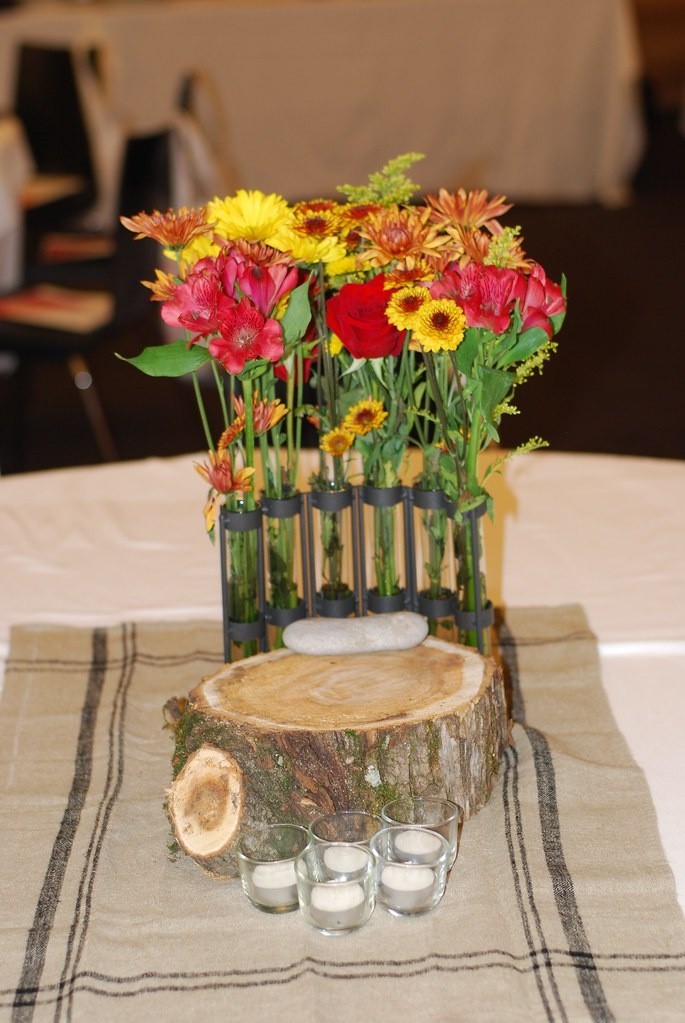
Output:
[235,822,313,915]
[294,842,376,938]
[369,825,451,919]
[307,809,386,845]
[381,796,459,872]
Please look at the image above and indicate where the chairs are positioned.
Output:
[0,38,217,465]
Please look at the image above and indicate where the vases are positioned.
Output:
[217,485,499,662]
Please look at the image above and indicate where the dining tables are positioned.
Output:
[1,443,685,1021]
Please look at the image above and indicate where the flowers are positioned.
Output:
[118,156,575,654]
